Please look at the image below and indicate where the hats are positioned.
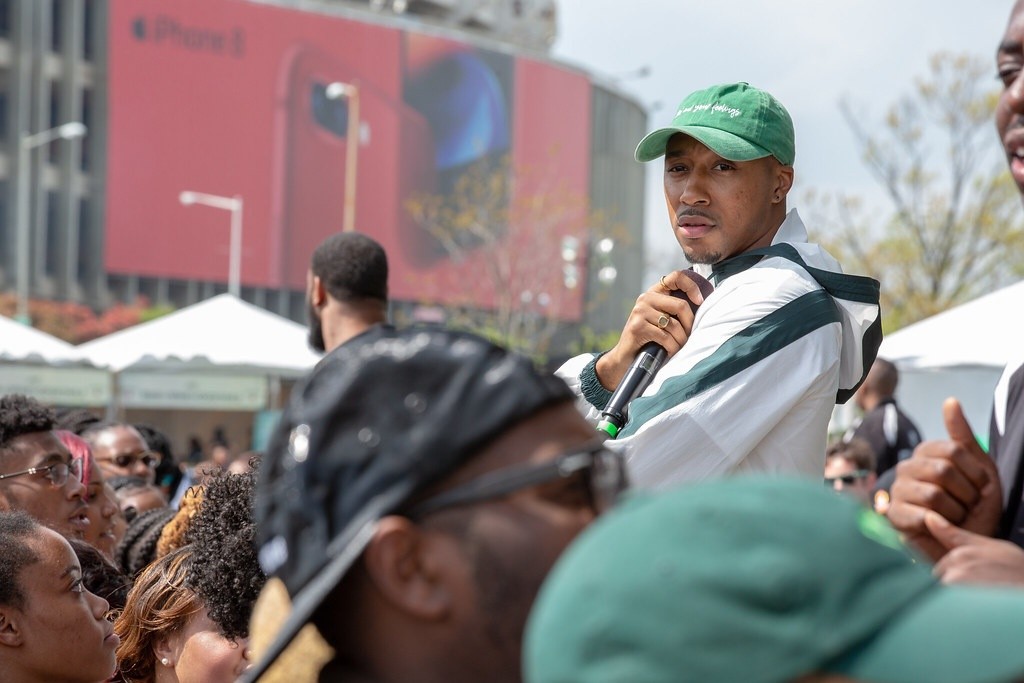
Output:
[634,82,796,165]
[231,324,551,683]
[520,470,1024,683]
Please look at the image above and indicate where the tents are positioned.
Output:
[1,314,118,424]
[831,280,1023,442]
[77,293,322,425]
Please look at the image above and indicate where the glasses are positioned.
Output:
[87,450,160,470]
[398,447,626,515]
[824,471,871,488]
[3,459,83,485]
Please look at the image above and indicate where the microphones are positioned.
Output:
[596,271,714,439]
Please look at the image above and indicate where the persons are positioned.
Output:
[235,325,596,681]
[827,439,878,495]
[308,231,388,353]
[556,82,882,495]
[0,394,268,682]
[854,357,921,474]
[889,0,1024,588]
[520,472,1024,683]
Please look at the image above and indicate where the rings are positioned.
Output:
[658,314,670,329]
[660,276,670,290]
